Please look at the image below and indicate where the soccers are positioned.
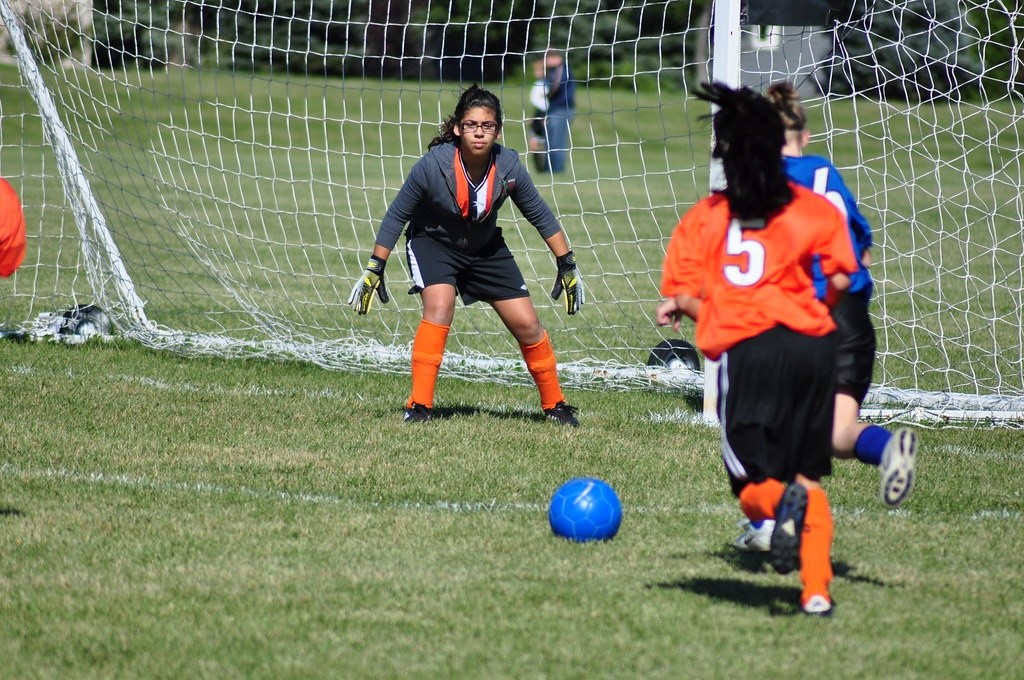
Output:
[548,476,624,542]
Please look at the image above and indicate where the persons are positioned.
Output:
[656,80,918,618]
[348,84,585,428]
[529,47,576,174]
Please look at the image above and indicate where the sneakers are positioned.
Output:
[877,427,918,506]
[544,402,579,428]
[404,402,431,421]
[735,517,774,552]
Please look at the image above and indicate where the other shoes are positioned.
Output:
[771,483,807,574]
[802,592,832,616]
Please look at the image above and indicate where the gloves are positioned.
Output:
[347,256,389,315]
[551,251,584,315]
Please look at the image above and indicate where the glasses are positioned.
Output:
[461,120,499,135]
